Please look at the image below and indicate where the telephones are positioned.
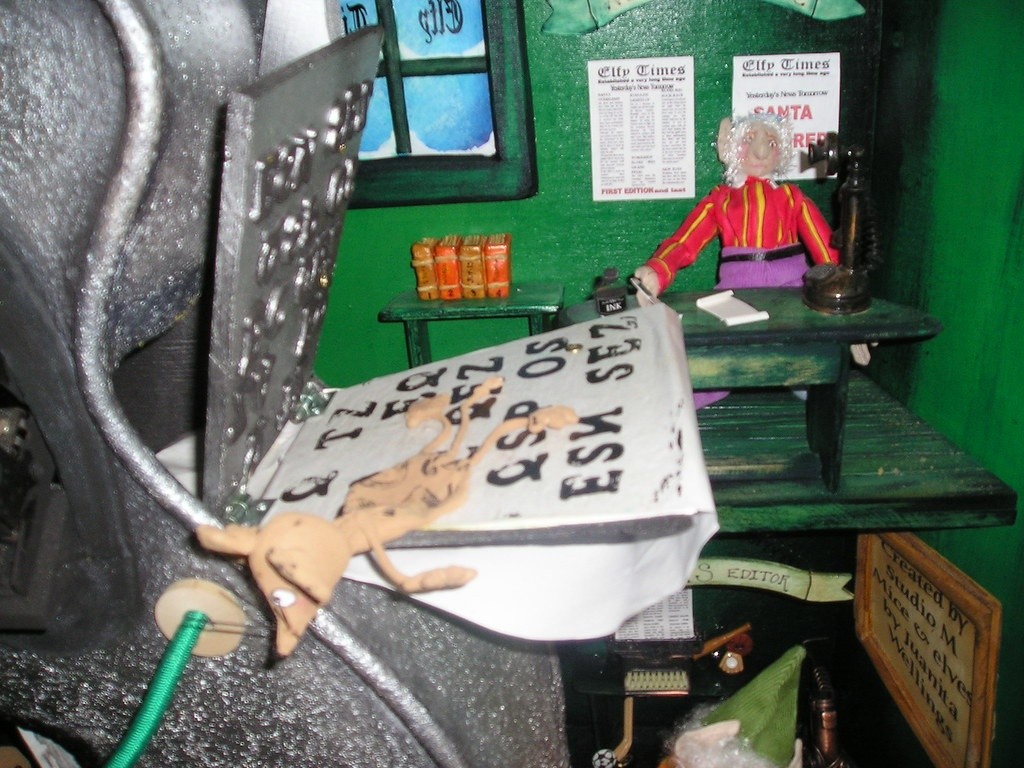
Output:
[802,132,874,316]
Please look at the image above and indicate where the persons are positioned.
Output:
[638,114,841,411]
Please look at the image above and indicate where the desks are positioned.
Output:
[560,289,1024,679]
[375,290,563,361]
